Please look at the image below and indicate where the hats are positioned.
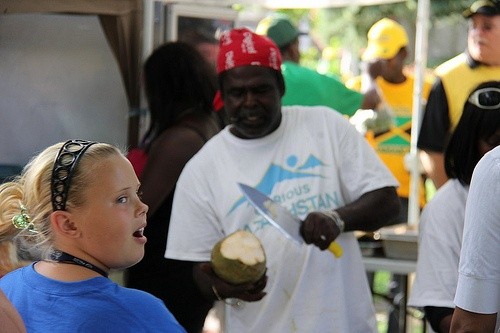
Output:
[256,15,308,46]
[462,0,500,19]
[361,17,409,62]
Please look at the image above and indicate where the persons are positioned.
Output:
[164,28,401,333]
[0,41,223,333]
[254,0,500,333]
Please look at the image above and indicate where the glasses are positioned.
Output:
[468,88,500,108]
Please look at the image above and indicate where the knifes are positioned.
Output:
[238,182,343,260]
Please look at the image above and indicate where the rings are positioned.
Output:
[320,234,326,241]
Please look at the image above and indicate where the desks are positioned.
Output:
[360,257,420,333]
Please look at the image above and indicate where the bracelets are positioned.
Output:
[211,283,243,306]
[320,210,345,233]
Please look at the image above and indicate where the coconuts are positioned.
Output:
[210,230,267,283]
